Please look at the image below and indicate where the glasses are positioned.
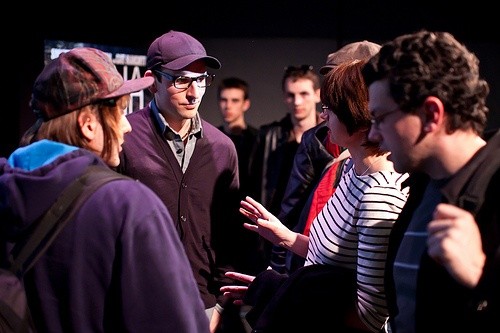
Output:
[370,109,400,126]
[321,104,330,112]
[284,64,314,73]
[156,70,215,89]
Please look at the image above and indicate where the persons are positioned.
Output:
[247,66,325,277]
[0,47,212,333]
[219,58,411,333]
[362,31,500,333]
[209,78,260,297]
[116,30,240,333]
[271,40,382,274]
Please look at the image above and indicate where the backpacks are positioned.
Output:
[0,167,132,333]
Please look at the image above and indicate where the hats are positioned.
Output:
[31,47,155,121]
[320,40,382,76]
[147,31,221,71]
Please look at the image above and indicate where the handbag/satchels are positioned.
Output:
[246,263,355,332]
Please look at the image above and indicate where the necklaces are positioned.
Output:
[360,157,380,178]
